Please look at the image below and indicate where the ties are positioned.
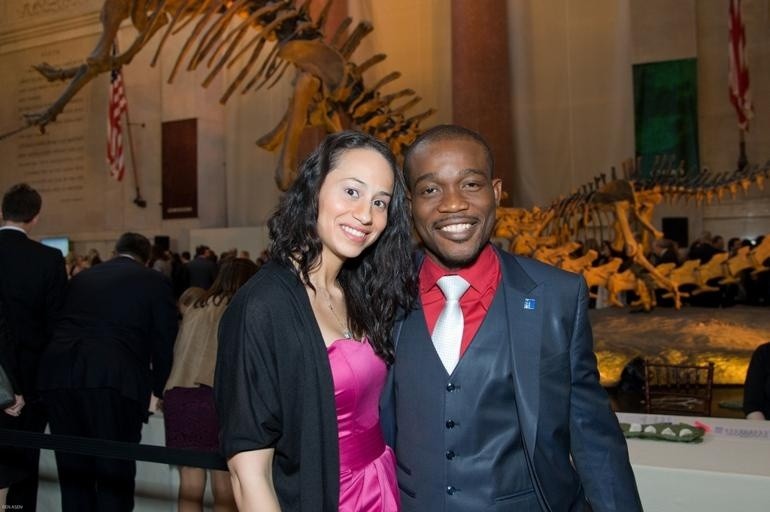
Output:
[431,275,470,375]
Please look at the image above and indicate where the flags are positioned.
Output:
[102,43,131,181]
[724,0,757,134]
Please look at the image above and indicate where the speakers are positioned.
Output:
[160,118,227,223]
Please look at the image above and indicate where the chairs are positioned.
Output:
[643,358,715,416]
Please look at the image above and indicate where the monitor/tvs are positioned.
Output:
[38,237,69,261]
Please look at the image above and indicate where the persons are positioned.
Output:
[561,231,770,307]
[744,341,770,421]
